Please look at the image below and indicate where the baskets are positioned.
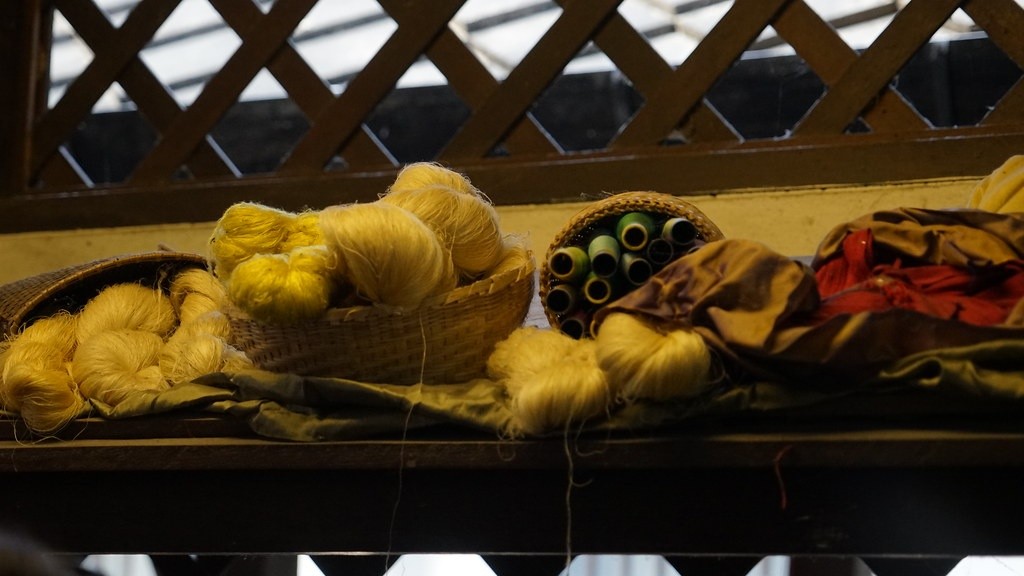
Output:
[230,245,537,385]
[0,249,218,339]
[538,192,725,341]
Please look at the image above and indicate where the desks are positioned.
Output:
[0,426,1024,576]
